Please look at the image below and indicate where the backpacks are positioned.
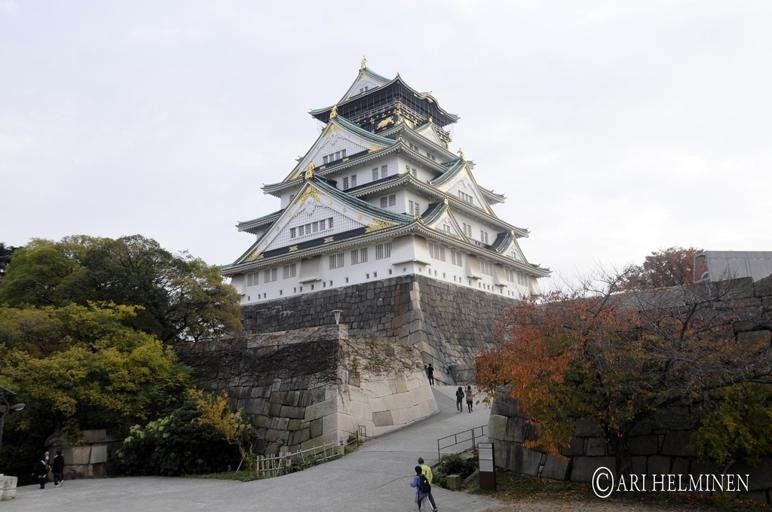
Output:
[416,474,430,493]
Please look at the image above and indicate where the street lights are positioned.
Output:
[331,310,344,325]
[701,272,710,284]
[693,253,706,285]
[0,403,26,447]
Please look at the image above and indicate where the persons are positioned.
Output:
[465,385,473,413]
[418,457,433,487]
[52,451,64,488]
[31,451,50,490]
[455,386,464,412]
[410,466,438,512]
[424,365,429,378]
[428,364,435,386]
